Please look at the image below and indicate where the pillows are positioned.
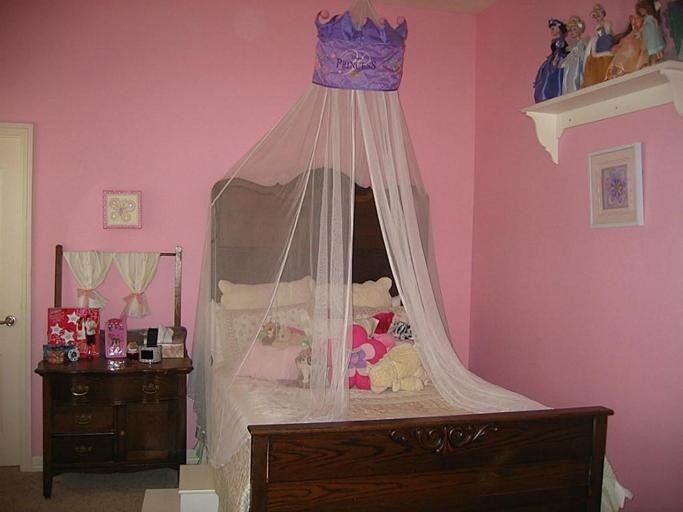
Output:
[214,272,391,380]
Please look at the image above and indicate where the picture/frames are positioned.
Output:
[586,140,645,227]
[104,188,142,230]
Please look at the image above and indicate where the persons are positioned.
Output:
[85,314,100,356]
[75,316,88,355]
[529,0,668,104]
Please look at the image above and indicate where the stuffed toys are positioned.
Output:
[259,321,280,346]
[368,342,433,394]
[294,347,316,390]
[271,327,303,348]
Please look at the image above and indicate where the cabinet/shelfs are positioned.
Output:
[34,345,194,499]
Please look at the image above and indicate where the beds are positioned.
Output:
[203,168,616,512]
